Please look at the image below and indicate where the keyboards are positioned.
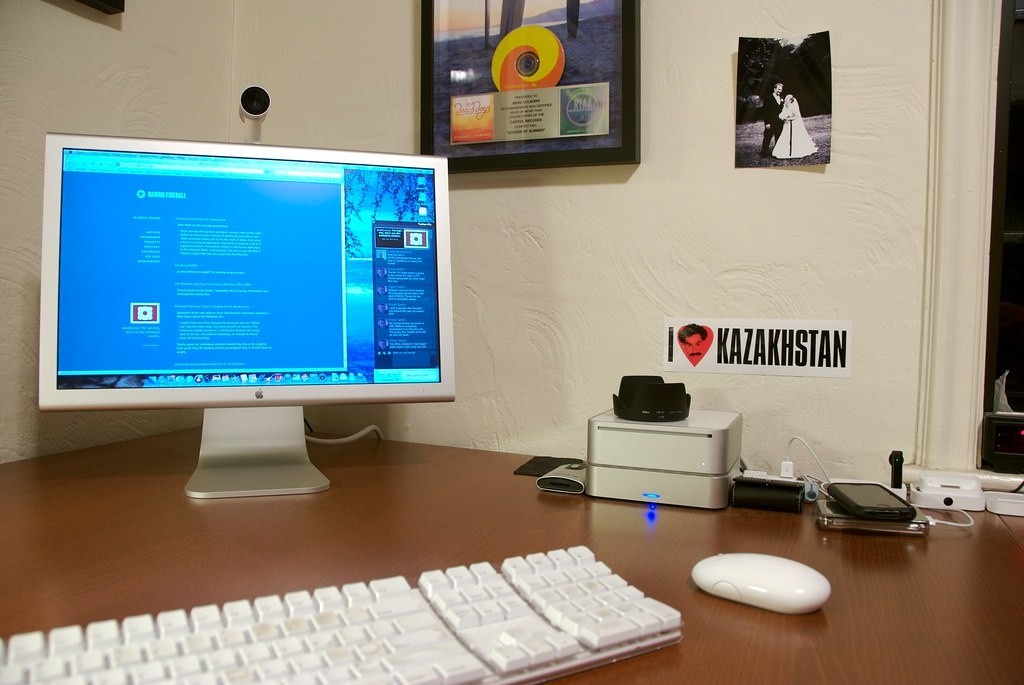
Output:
[0,546,684,685]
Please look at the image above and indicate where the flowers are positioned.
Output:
[778,112,790,121]
[781,99,783,102]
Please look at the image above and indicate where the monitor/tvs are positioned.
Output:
[39,129,456,499]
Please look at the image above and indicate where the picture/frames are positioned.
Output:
[421,0,642,173]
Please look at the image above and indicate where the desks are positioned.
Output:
[0,425,1023,685]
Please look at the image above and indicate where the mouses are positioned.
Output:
[690,553,832,614]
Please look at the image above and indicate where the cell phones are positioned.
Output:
[827,483,917,522]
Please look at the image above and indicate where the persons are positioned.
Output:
[679,323,708,360]
[759,83,818,158]
